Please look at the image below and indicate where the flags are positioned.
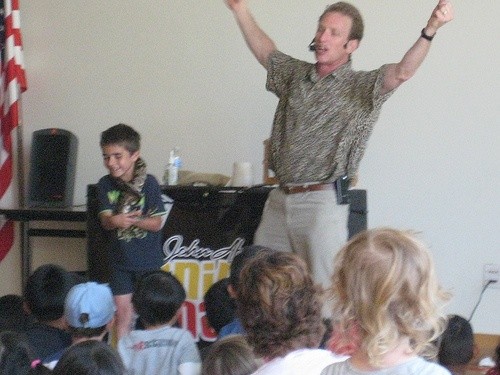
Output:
[0,0,27,262]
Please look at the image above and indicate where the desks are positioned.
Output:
[88,184,369,357]
[0,208,86,295]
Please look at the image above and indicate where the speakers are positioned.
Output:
[25,128,79,208]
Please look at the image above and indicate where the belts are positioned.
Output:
[278,183,337,194]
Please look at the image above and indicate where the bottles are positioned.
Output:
[168,147,181,185]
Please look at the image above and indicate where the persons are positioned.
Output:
[0,229,478,375]
[226,0,455,321]
[93,123,166,342]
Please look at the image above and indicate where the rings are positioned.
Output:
[442,10,448,16]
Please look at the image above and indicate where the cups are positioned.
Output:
[233,161,253,188]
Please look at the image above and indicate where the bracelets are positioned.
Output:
[421,28,437,42]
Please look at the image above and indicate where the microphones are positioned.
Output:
[343,38,351,49]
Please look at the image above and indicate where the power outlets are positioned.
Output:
[484,267,500,289]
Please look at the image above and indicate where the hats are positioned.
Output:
[62,283,115,329]
